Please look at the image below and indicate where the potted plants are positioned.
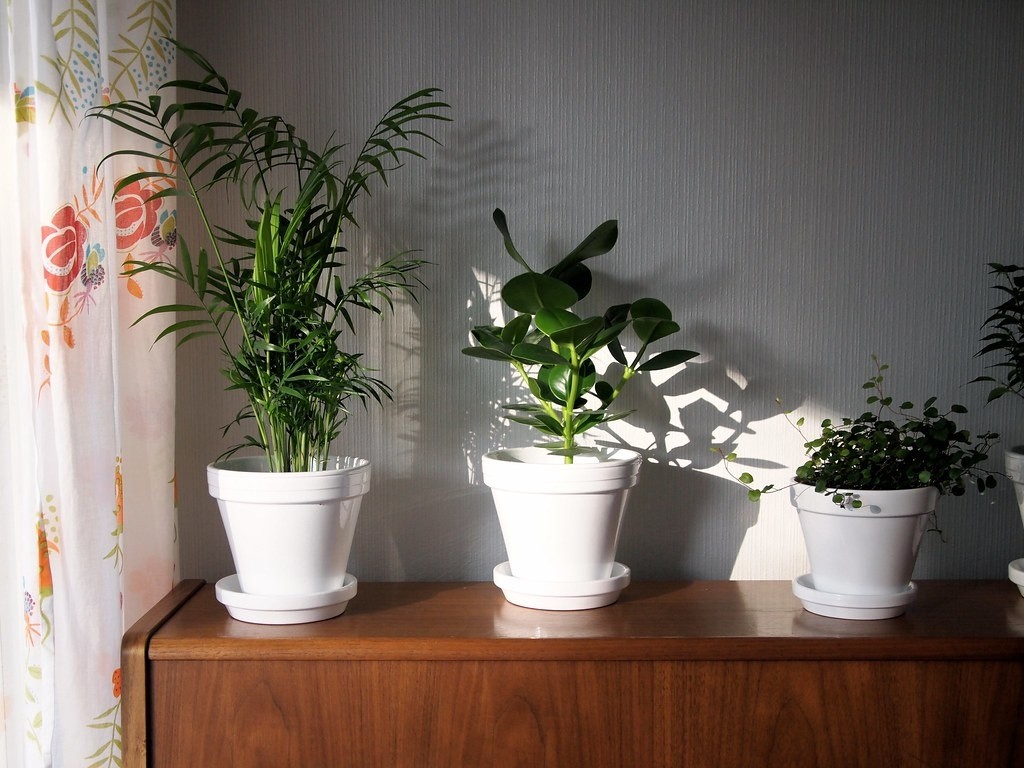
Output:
[960,255,1024,623]
[714,348,1008,618]
[461,207,699,610]
[76,35,453,624]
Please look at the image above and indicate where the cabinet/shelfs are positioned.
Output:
[120,579,1024,768]
